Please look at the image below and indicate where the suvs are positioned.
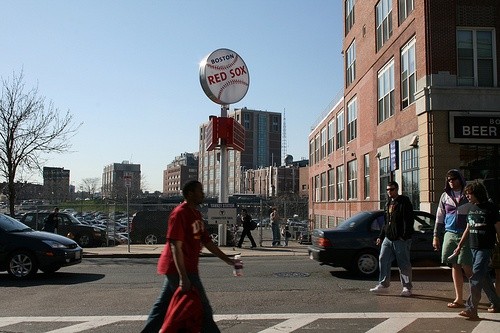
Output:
[20,211,106,249]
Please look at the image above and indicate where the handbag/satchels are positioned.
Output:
[250,220,257,230]
[157,285,203,333]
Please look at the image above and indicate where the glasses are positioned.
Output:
[386,188,396,191]
[447,177,458,182]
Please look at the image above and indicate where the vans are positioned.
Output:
[129,210,176,245]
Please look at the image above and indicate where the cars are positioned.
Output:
[306,207,445,279]
[64,209,133,246]
[0,212,84,282]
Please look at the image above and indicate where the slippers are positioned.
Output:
[448,300,466,308]
[488,307,496,312]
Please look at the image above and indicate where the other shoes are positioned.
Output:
[238,244,241,248]
[458,310,479,318]
[250,244,256,248]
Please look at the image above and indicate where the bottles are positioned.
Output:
[232,255,244,277]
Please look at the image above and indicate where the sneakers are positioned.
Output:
[399,287,412,297]
[370,285,388,294]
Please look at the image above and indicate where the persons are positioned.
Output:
[453,183,500,318]
[47,207,59,228]
[269,208,283,246]
[235,209,257,248]
[139,179,244,333]
[432,169,474,307]
[370,181,414,296]
[281,223,288,246]
